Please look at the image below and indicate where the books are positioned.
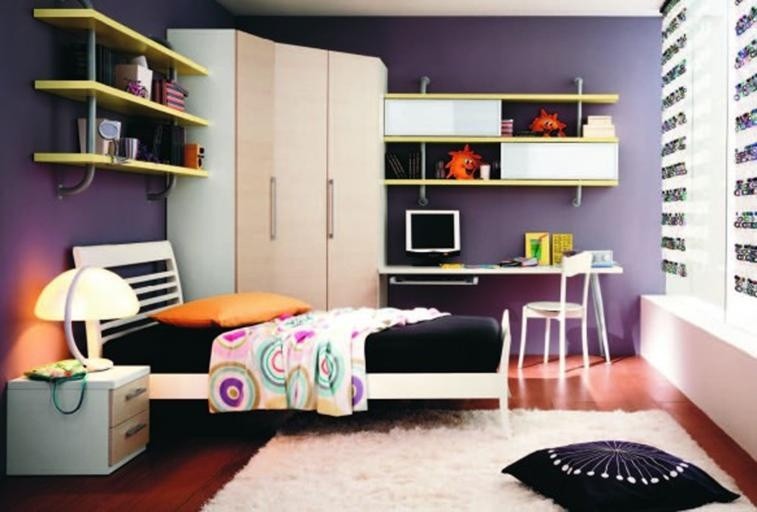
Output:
[386,150,446,179]
[71,42,112,86]
[148,125,185,165]
[152,79,161,103]
[487,159,500,179]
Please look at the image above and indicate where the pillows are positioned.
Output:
[500,442,741,512]
[147,292,315,329]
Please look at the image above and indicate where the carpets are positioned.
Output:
[199,406,757,511]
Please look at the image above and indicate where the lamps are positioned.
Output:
[34,266,141,373]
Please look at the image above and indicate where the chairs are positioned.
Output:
[518,249,593,373]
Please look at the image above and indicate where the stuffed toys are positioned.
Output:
[447,146,479,179]
[531,108,568,137]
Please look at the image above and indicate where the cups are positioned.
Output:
[480,165,491,180]
[121,137,140,160]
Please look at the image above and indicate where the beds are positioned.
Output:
[73,240,511,412]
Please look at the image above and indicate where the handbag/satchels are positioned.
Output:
[23,359,86,414]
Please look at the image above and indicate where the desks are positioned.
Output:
[380,264,623,366]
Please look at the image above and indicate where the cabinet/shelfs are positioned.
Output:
[640,295,757,462]
[32,7,210,177]
[328,50,388,311]
[382,93,619,187]
[167,29,328,312]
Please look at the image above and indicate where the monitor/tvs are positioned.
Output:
[405,209,461,266]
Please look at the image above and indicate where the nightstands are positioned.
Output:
[6,364,151,477]
